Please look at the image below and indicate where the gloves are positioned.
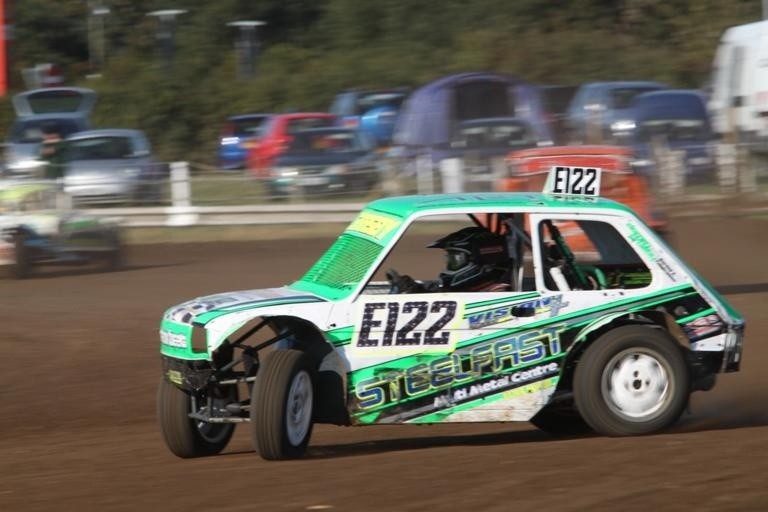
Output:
[397,275,423,292]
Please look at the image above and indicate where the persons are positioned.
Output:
[398,225,511,293]
[36,122,68,179]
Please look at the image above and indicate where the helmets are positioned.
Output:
[426,227,512,292]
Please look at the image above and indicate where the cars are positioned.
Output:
[0,87,164,271]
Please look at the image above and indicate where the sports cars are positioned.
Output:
[160,192,745,461]
[475,144,664,269]
